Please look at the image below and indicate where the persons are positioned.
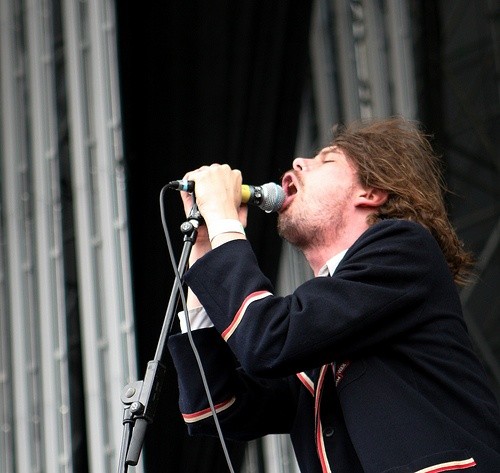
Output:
[167,114,499,473]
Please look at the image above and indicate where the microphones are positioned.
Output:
[170,180,286,213]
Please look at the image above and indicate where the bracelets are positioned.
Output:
[206,219,246,242]
